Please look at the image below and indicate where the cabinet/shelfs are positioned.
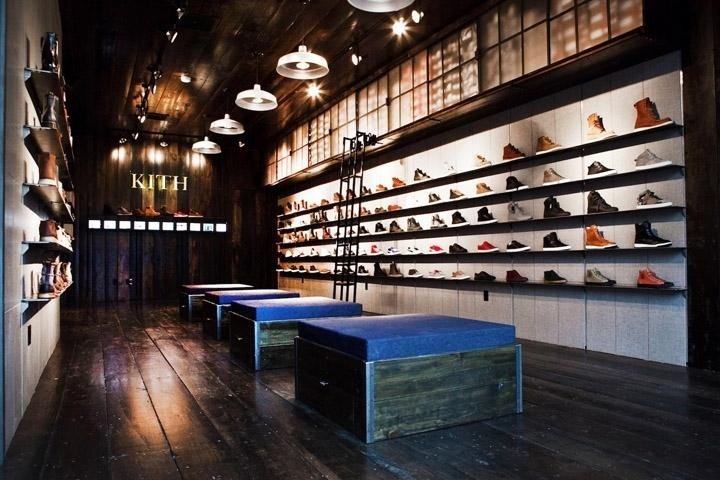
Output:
[88,216,227,232]
[274,121,687,296]
[22,67,75,302]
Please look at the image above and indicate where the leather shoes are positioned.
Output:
[376,207,387,213]
[389,205,402,211]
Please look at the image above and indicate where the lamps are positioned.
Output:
[347,0,416,12]
[149,83,157,94]
[191,123,221,154]
[235,50,277,112]
[208,96,244,135]
[166,30,177,44]
[275,4,329,79]
[351,52,362,66]
[137,113,146,124]
[132,132,139,140]
[411,8,424,23]
[177,8,185,19]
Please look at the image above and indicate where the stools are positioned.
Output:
[201,290,300,341]
[229,299,364,371]
[178,284,254,323]
[293,315,522,444]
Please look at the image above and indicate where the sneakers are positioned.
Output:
[103,206,115,216]
[638,190,673,209]
[407,246,423,255]
[536,136,562,155]
[188,209,204,217]
[449,244,467,253]
[300,221,307,226]
[586,269,616,285]
[408,269,423,278]
[132,209,146,216]
[451,272,470,280]
[429,270,446,279]
[362,187,370,196]
[361,207,370,215]
[449,189,467,201]
[504,144,526,162]
[310,266,319,273]
[375,223,389,234]
[429,195,444,205]
[587,162,617,179]
[544,198,571,217]
[116,207,132,215]
[385,247,401,255]
[544,270,567,283]
[441,162,457,177]
[359,226,371,236]
[478,241,500,252]
[174,211,188,217]
[320,269,331,274]
[587,190,619,214]
[146,207,160,216]
[290,265,298,272]
[334,268,354,275]
[284,265,290,272]
[506,240,531,252]
[508,202,532,221]
[471,155,491,169]
[635,149,672,170]
[322,200,329,205]
[429,246,446,255]
[452,212,469,227]
[159,207,174,216]
[430,215,447,229]
[282,205,293,215]
[376,185,387,193]
[334,193,345,203]
[276,265,283,272]
[371,245,384,255]
[478,207,498,225]
[38,219,57,241]
[474,271,496,281]
[57,225,72,246]
[636,269,675,288]
[543,168,571,185]
[298,266,307,273]
[407,218,423,231]
[390,221,403,232]
[506,177,528,192]
[358,249,366,256]
[392,178,407,188]
[476,184,496,198]
[310,204,319,208]
[506,270,528,281]
[293,248,356,257]
[346,190,358,200]
[543,232,571,251]
[301,200,306,211]
[334,230,356,237]
[285,250,292,258]
[358,266,370,276]
[414,169,432,184]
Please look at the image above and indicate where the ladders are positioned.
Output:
[333,130,367,302]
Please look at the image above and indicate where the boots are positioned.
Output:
[335,207,345,221]
[323,225,331,239]
[309,228,317,240]
[389,264,403,278]
[54,263,65,291]
[292,202,299,213]
[586,113,617,141]
[37,151,57,185]
[374,262,386,277]
[41,31,58,72]
[310,210,328,224]
[634,98,672,132]
[41,93,60,128]
[634,222,672,248]
[38,263,55,299]
[585,225,618,249]
[62,262,72,286]
[282,231,306,244]
